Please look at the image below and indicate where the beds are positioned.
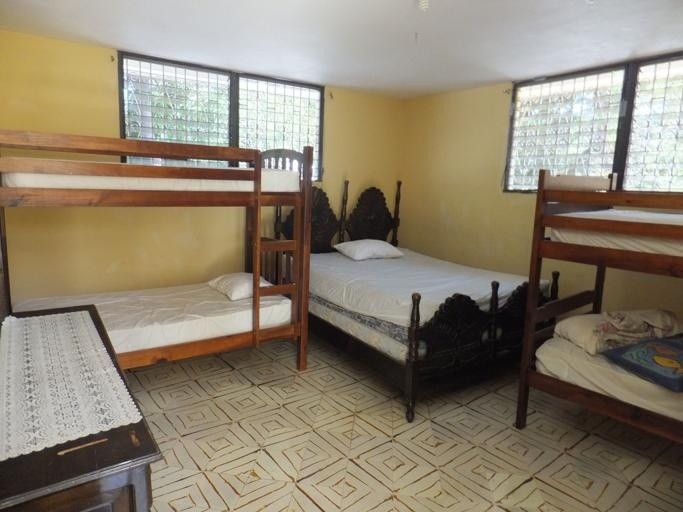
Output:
[0,127,314,371]
[515,171,683,445]
[271,178,560,421]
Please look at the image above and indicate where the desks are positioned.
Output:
[0,304,161,510]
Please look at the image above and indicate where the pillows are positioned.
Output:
[206,268,274,300]
[332,238,404,261]
[553,309,683,392]
